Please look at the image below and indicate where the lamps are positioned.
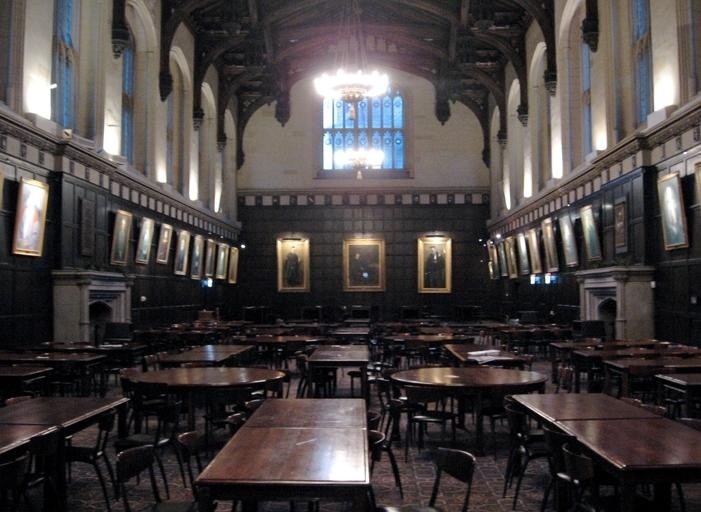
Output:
[307,0,392,179]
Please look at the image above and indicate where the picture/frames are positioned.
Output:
[418,236,452,295]
[342,238,386,293]
[12,177,240,284]
[487,171,691,280]
[275,232,311,293]
